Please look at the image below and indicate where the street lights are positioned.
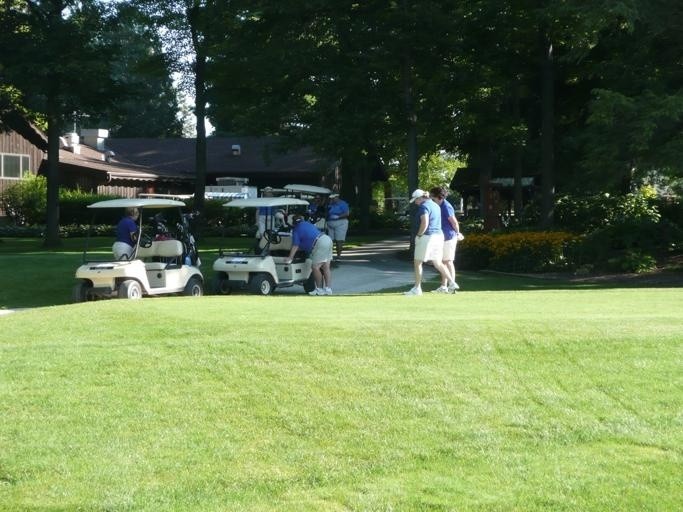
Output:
[255,229,260,239]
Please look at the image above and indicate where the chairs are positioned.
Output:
[323,286,332,295]
[430,287,448,295]
[448,284,460,293]
[403,288,422,296]
[336,256,342,259]
[308,288,325,296]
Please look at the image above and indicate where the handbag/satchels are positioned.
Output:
[417,233,423,237]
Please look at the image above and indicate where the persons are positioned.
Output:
[429,186,464,292]
[283,213,333,296]
[112,208,139,261]
[326,192,349,262]
[255,186,279,240]
[309,194,321,205]
[403,188,460,294]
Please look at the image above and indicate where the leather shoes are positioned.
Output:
[330,214,339,219]
[456,232,464,241]
[415,235,423,244]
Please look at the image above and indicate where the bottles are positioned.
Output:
[264,186,272,193]
[409,188,430,204]
[292,216,302,223]
[328,191,340,198]
